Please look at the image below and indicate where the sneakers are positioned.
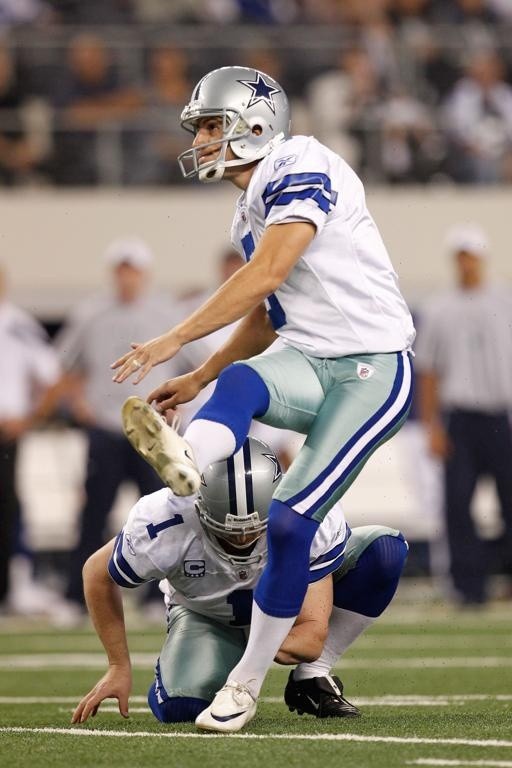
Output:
[285,669,361,717]
[122,396,201,496]
[195,679,260,733]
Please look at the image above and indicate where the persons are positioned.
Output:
[107,67,416,733]
[1,298,89,616]
[59,238,193,611]
[1,4,510,184]
[418,231,512,606]
[69,436,411,727]
[179,244,304,456]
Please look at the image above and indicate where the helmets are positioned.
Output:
[178,66,291,182]
[195,435,281,565]
